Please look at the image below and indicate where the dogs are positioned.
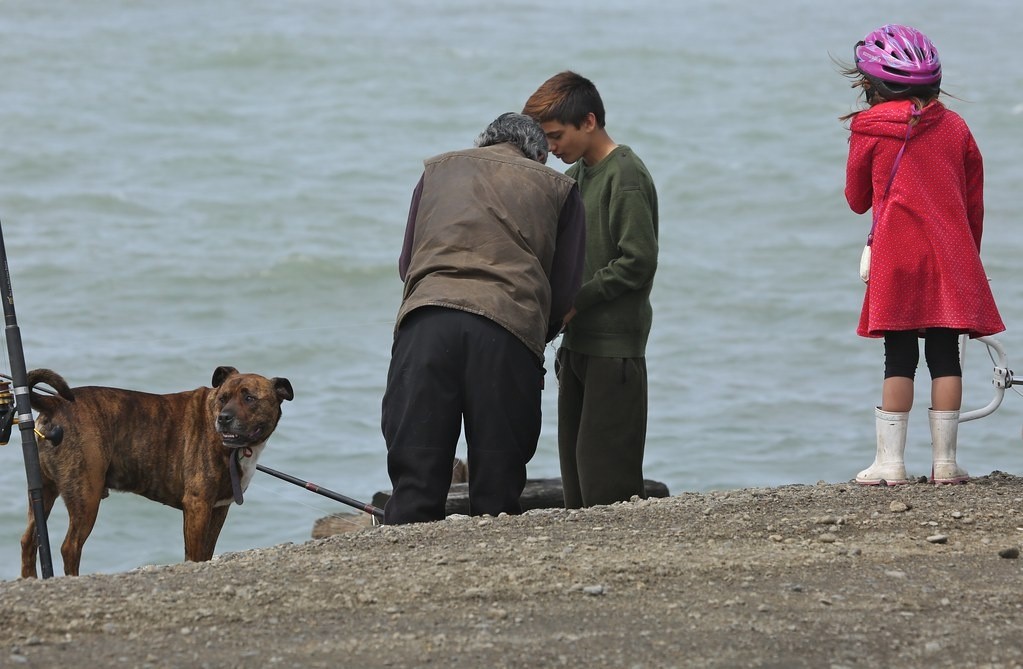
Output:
[20,365,294,578]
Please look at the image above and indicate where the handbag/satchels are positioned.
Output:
[860,244,871,282]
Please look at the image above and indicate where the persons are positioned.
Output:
[521,72,660,510]
[841,24,1006,486]
[382,111,588,526]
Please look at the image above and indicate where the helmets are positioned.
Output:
[855,24,942,84]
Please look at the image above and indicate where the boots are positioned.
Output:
[928,408,969,482]
[855,407,909,485]
